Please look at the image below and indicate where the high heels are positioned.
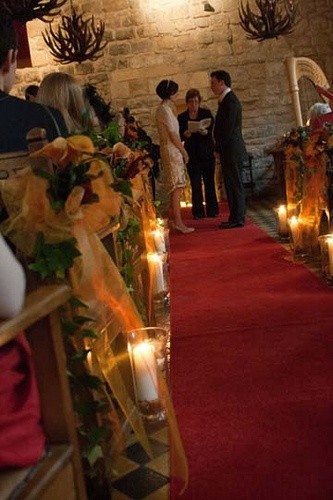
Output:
[170,224,195,234]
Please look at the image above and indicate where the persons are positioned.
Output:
[25,85,39,102]
[0,0,69,155]
[0,236,48,471]
[155,79,195,233]
[35,72,86,135]
[307,86,333,166]
[210,70,249,228]
[177,89,219,220]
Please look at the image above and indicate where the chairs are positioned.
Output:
[242,155,255,201]
[0,128,155,500]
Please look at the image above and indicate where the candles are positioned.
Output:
[289,216,302,249]
[132,219,166,402]
[327,238,333,276]
[277,204,286,234]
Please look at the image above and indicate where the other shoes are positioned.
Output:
[208,214,217,217]
[219,221,243,228]
[194,216,203,219]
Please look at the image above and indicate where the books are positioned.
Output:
[188,118,211,133]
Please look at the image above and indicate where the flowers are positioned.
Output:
[26,107,156,468]
[281,121,332,215]
